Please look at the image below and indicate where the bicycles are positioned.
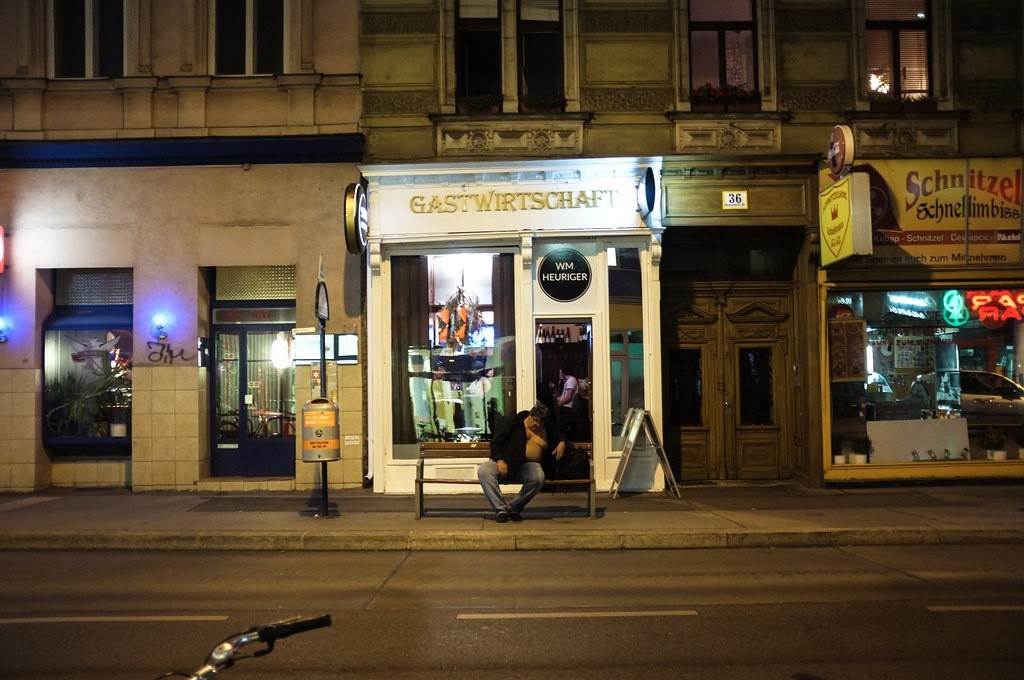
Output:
[417,424,452,442]
[155,614,332,680]
[218,409,296,439]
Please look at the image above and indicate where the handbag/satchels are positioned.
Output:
[541,442,590,481]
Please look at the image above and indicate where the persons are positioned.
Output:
[477,367,591,523]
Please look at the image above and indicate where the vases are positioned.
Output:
[692,102,725,112]
[728,103,760,113]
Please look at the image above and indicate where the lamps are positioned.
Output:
[961,448,968,457]
[944,449,949,458]
[912,450,920,459]
[928,449,936,459]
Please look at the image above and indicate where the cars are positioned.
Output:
[926,370,1024,415]
[48,391,132,437]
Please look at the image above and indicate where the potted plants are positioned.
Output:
[457,95,503,116]
[981,430,1007,460]
[522,94,567,114]
[832,434,845,464]
[1015,430,1024,459]
[849,431,871,464]
[869,93,938,113]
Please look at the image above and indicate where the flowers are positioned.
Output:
[688,82,761,105]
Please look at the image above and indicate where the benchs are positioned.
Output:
[415,442,596,520]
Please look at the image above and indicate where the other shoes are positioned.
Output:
[508,509,523,522]
[496,513,507,522]
